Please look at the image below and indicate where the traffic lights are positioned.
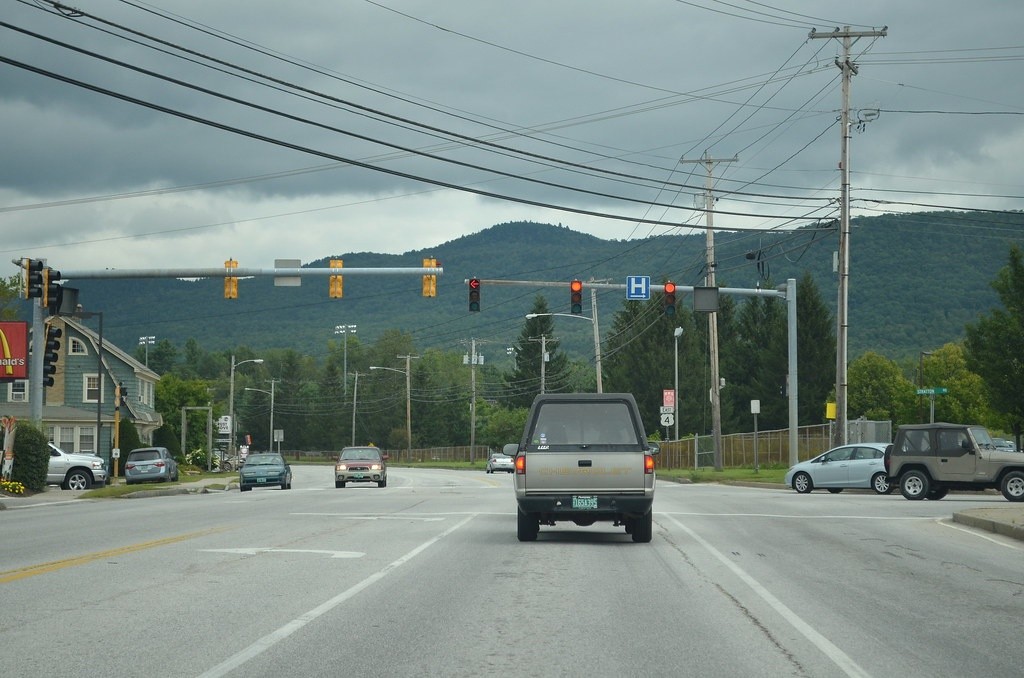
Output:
[44,327,62,387]
[665,283,676,316]
[468,279,480,312]
[48,270,61,307]
[571,280,582,315]
[29,260,43,297]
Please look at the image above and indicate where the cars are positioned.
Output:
[332,446,390,488]
[990,437,1024,453]
[502,393,662,543]
[236,452,294,491]
[45,442,109,490]
[484,453,514,474]
[125,447,178,484]
[784,442,908,495]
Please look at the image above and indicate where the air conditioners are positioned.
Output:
[138,395,144,402]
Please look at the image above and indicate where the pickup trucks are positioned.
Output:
[884,422,1024,502]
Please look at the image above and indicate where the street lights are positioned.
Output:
[139,334,156,368]
[526,313,604,393]
[228,359,264,453]
[334,323,357,395]
[370,366,412,462]
[347,371,365,446]
[244,387,275,453]
[674,326,683,441]
